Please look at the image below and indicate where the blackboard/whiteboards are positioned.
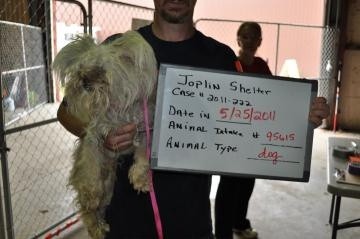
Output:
[150,64,318,183]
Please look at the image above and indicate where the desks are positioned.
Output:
[328,137,360,239]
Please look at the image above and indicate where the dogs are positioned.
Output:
[50,30,158,239]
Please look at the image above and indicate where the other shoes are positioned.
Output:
[232,229,257,239]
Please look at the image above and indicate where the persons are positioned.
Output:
[58,0,331,239]
[214,22,273,239]
